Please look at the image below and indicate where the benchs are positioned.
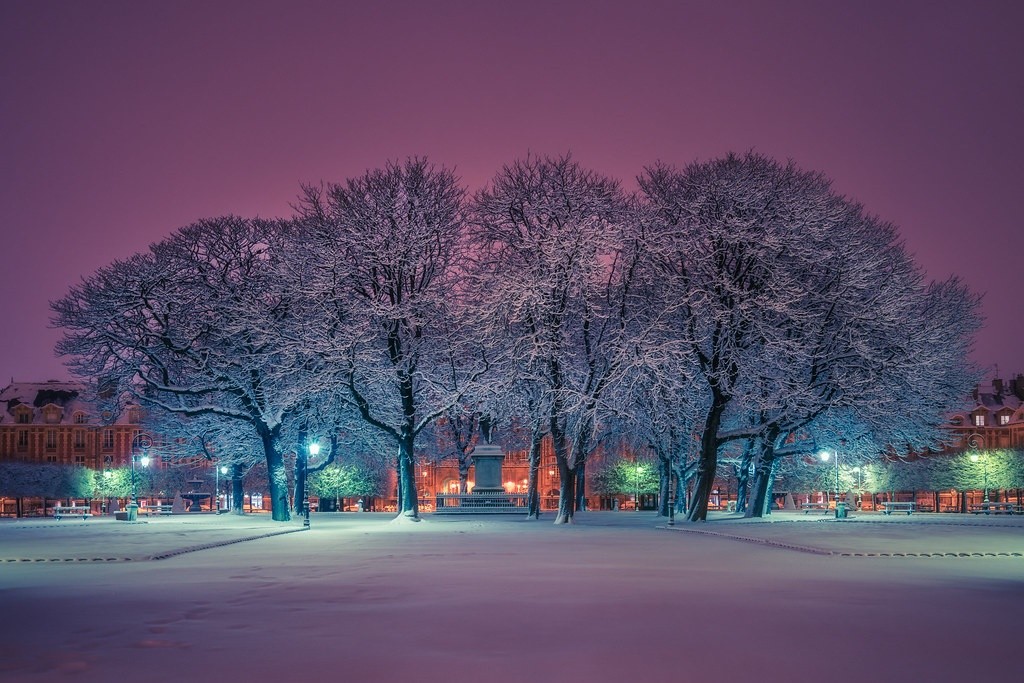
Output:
[143,505,173,517]
[970,504,1015,515]
[878,502,916,515]
[802,503,831,514]
[52,506,93,522]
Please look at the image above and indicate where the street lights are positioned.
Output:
[215,465,228,515]
[101,455,112,516]
[820,447,839,518]
[126,433,154,521]
[967,433,990,515]
[302,433,320,527]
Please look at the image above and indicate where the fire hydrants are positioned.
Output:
[834,502,851,517]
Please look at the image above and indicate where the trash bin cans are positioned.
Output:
[126,504,138,521]
[837,502,846,518]
